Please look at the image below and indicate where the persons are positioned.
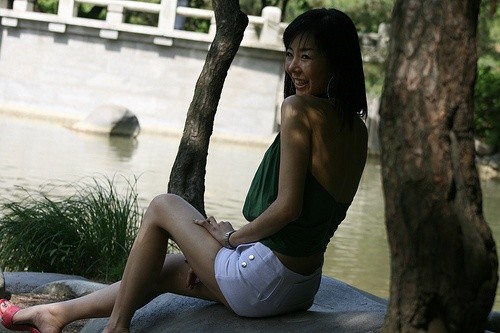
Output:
[0,7,368,333]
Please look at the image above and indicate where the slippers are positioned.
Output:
[0,298,41,333]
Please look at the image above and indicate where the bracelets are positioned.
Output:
[225,230,237,250]
